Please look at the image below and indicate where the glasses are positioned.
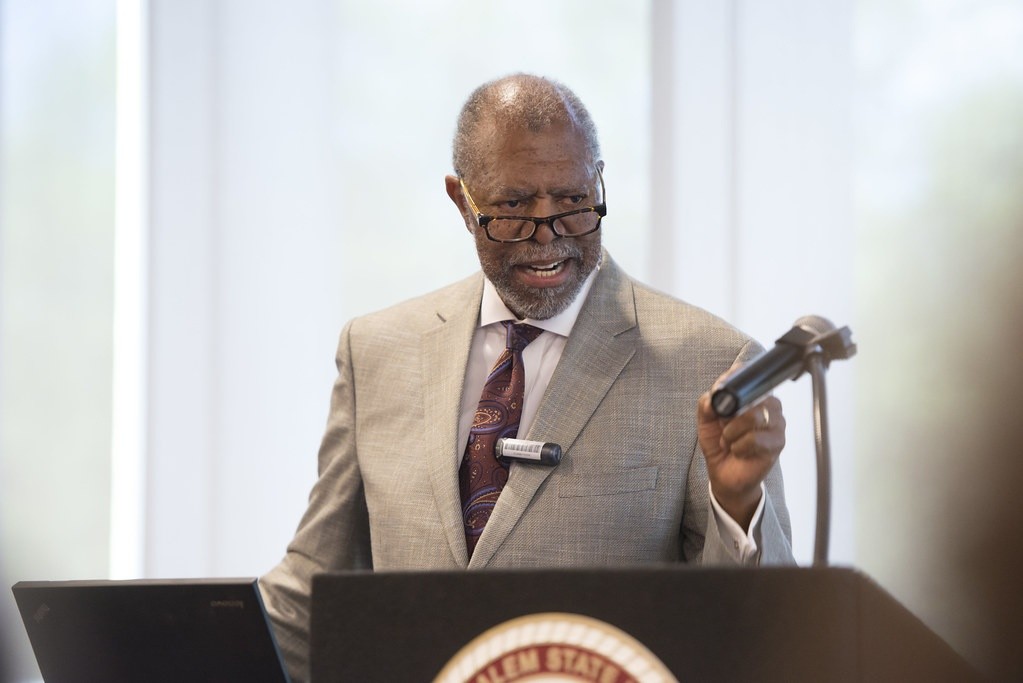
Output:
[460,165,607,244]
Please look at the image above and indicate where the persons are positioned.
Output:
[258,73,787,682]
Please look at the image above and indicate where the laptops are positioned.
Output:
[12,577,292,683]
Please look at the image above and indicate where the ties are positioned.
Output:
[458,320,544,562]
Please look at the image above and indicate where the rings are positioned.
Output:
[761,405,770,429]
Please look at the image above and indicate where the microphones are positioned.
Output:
[711,315,838,418]
